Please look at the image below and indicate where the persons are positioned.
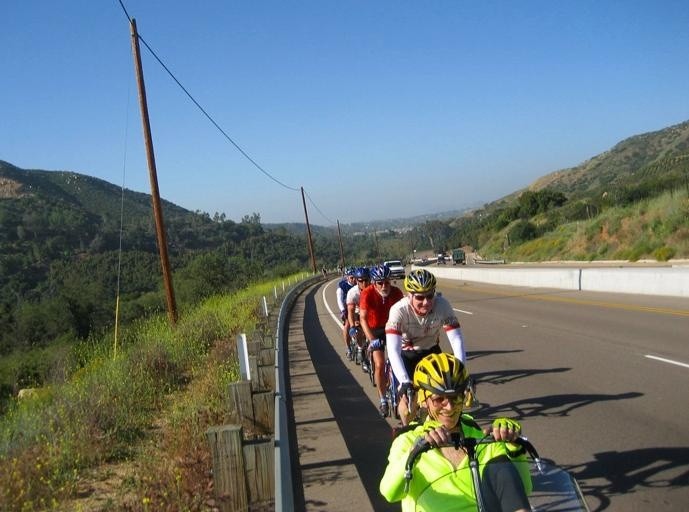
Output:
[376,350,535,511]
[318,263,477,424]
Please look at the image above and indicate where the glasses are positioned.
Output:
[416,292,434,300]
[378,281,389,285]
[433,397,463,407]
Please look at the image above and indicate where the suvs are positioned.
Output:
[383,260,406,280]
[437,257,446,266]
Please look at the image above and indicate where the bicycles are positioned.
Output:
[400,435,590,512]
[350,329,376,387]
[342,315,359,365]
[367,341,398,419]
[397,382,480,431]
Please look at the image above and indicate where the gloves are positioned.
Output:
[349,326,358,336]
[340,310,348,319]
[370,339,381,347]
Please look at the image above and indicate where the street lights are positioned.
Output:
[412,248,417,260]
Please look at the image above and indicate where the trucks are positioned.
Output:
[452,249,466,266]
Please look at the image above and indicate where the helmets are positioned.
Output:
[404,269,436,293]
[344,266,392,284]
[414,353,466,404]
